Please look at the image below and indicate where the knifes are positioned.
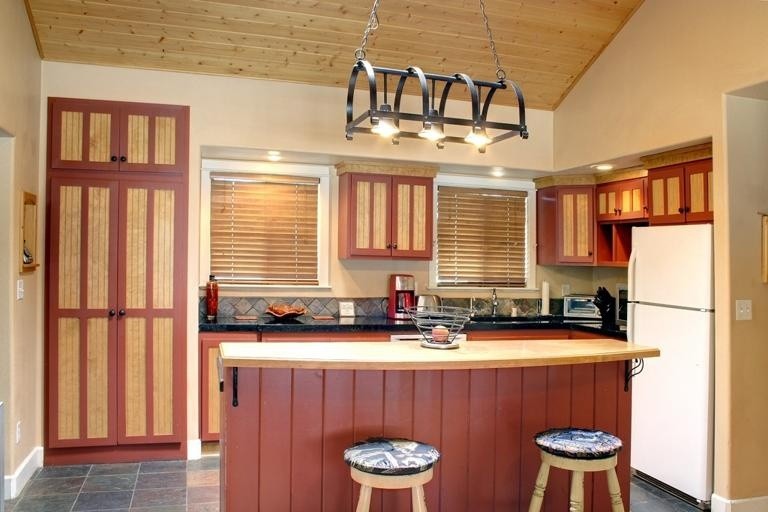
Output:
[593,285,609,306]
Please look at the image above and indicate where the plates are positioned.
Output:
[264,308,306,318]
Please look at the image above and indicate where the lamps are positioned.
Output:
[343,0,530,159]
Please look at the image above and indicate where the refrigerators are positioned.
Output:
[626,223,714,508]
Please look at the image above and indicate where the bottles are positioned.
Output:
[206,275,218,318]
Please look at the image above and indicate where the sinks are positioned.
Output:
[469,315,551,326]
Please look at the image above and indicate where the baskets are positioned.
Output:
[405,306,473,349]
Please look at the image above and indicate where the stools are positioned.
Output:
[525,422,628,512]
[341,435,442,512]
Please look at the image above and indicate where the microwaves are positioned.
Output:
[614,284,629,325]
[563,294,606,320]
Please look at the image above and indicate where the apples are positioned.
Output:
[432,325,448,342]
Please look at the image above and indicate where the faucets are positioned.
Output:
[491,287,500,317]
[468,295,477,317]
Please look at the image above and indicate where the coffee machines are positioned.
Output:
[388,274,415,319]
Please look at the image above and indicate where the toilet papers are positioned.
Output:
[540,280,550,316]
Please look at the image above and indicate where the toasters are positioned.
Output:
[415,294,443,319]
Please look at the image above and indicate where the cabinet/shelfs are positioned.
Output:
[259,320,328,340]
[464,323,521,340]
[595,160,652,270]
[638,142,715,228]
[568,326,626,340]
[195,327,260,459]
[518,321,573,341]
[40,169,191,468]
[532,172,596,268]
[334,158,439,262]
[42,92,193,184]
[324,321,391,342]
